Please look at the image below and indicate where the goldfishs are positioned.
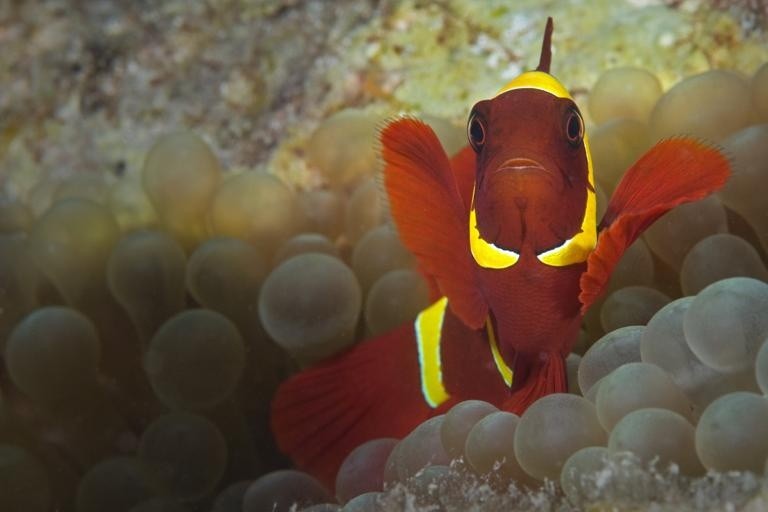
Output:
[269,18,737,503]
